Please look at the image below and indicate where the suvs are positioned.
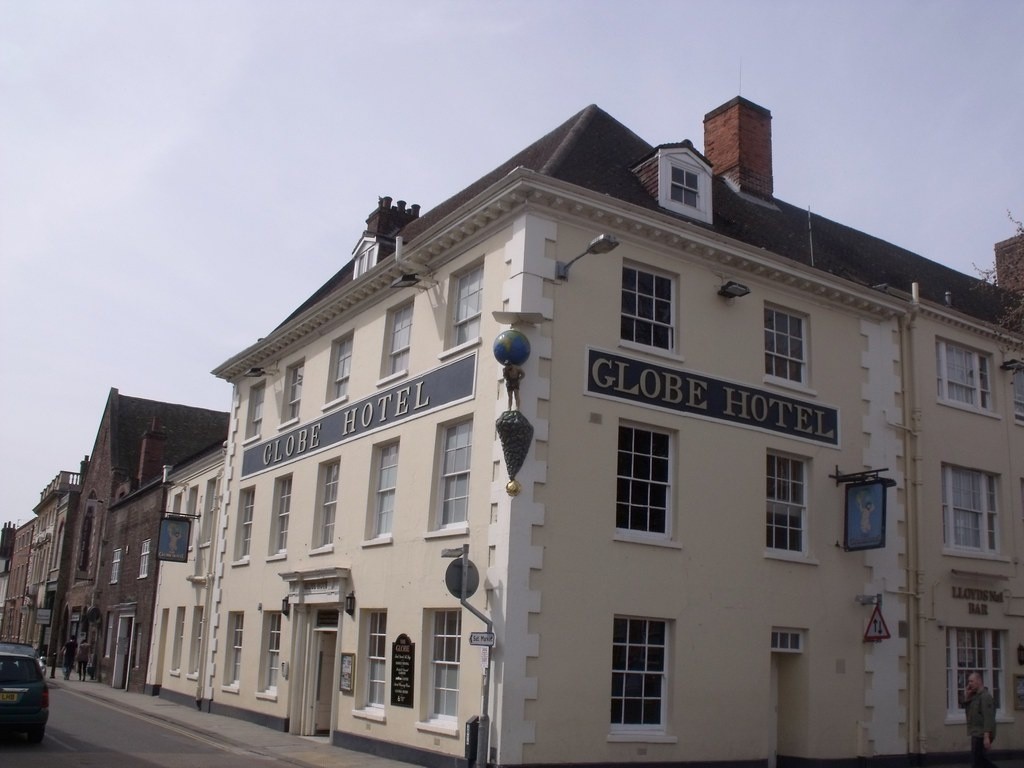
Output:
[0,642,50,744]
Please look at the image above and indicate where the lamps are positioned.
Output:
[281,593,289,616]
[554,232,620,283]
[241,367,279,377]
[344,590,355,616]
[389,271,438,293]
[718,279,749,299]
[1000,358,1024,370]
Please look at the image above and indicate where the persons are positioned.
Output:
[962,672,1001,768]
[503,360,525,411]
[60,635,78,680]
[75,637,91,682]
